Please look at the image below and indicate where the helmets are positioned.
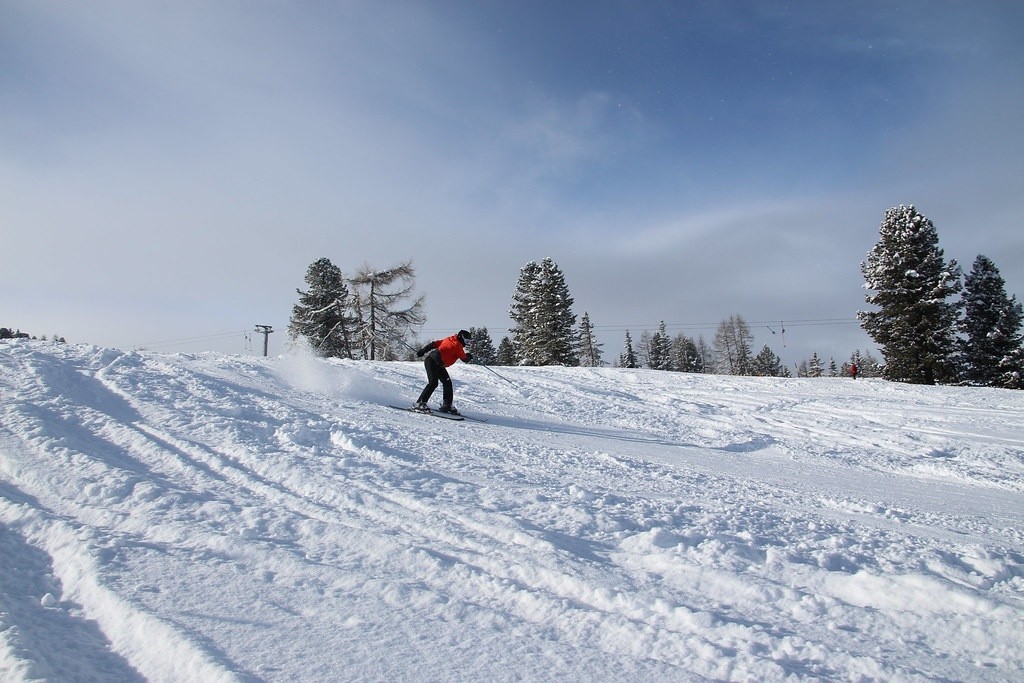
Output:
[458,330,473,347]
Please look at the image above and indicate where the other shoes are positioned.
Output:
[413,402,431,413]
[443,402,460,415]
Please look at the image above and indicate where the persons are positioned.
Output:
[411,330,472,415]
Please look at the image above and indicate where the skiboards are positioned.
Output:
[388,404,487,423]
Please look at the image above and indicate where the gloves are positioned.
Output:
[417,342,434,357]
[460,353,473,363]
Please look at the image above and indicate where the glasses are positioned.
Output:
[464,339,473,345]
